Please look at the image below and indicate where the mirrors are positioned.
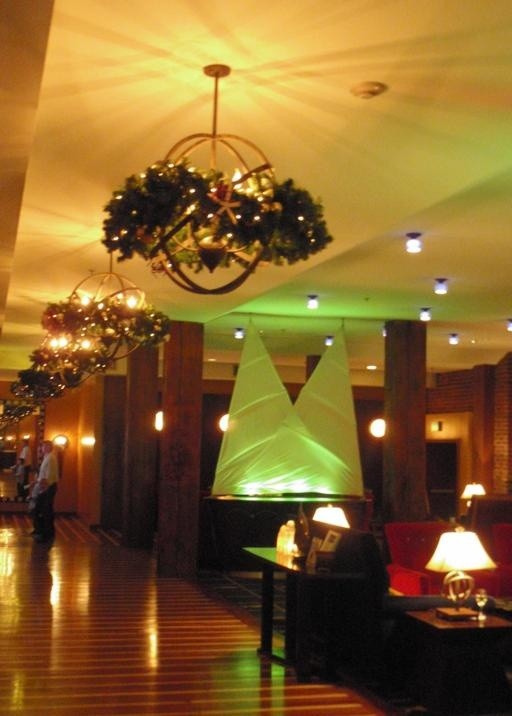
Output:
[0,401,48,504]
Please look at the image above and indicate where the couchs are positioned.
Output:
[384,517,512,600]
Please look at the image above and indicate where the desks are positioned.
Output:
[407,608,512,716]
[241,544,357,684]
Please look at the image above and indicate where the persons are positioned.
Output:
[10,439,32,502]
[27,440,59,543]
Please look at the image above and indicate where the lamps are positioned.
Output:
[1,229,167,427]
[424,525,497,622]
[101,64,334,295]
[234,231,512,349]
